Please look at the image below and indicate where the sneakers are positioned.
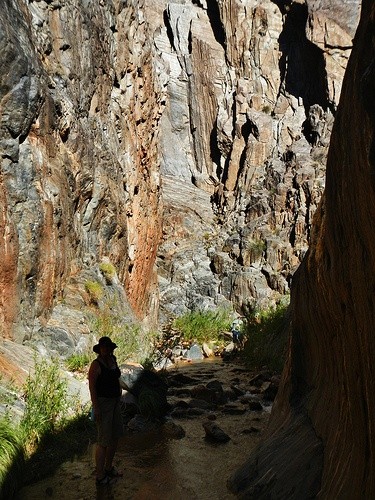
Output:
[104,466,123,477]
[96,476,117,487]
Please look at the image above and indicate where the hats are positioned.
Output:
[93,337,118,354]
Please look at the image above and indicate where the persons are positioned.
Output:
[232,326,241,351]
[88,336,124,495]
